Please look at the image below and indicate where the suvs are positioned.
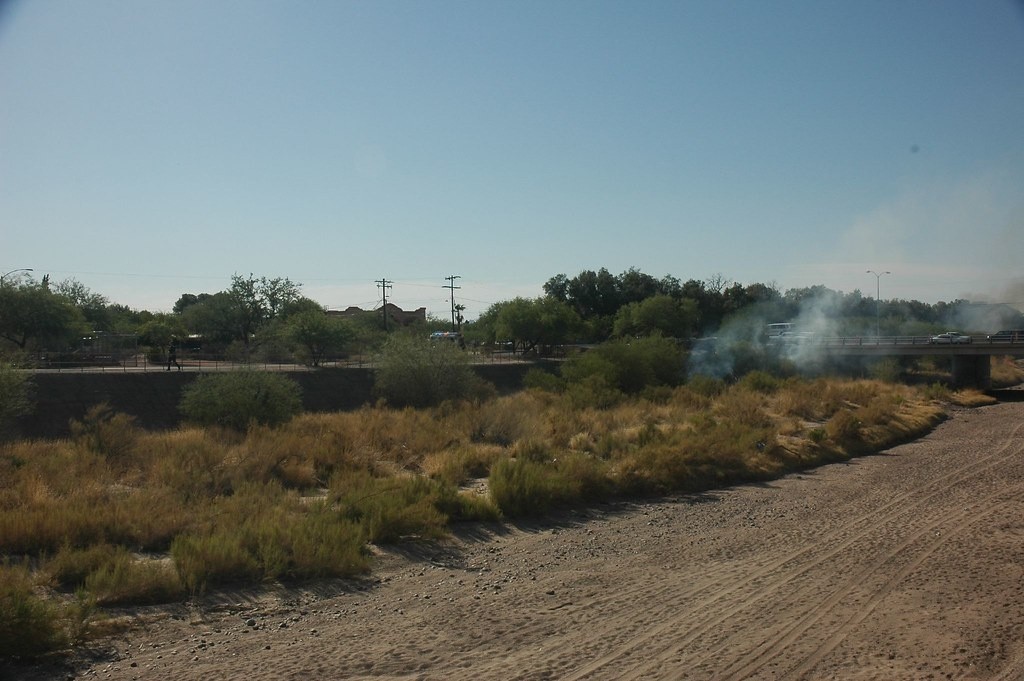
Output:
[946,331,972,341]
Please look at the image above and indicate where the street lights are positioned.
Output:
[867,269,891,338]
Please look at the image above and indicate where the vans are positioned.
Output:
[987,330,1024,339]
[768,323,797,334]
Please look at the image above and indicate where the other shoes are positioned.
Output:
[166,370,170,371]
[179,366,180,369]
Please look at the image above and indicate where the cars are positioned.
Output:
[933,334,968,344]
[771,332,807,344]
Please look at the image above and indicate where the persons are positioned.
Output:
[166,342,180,371]
